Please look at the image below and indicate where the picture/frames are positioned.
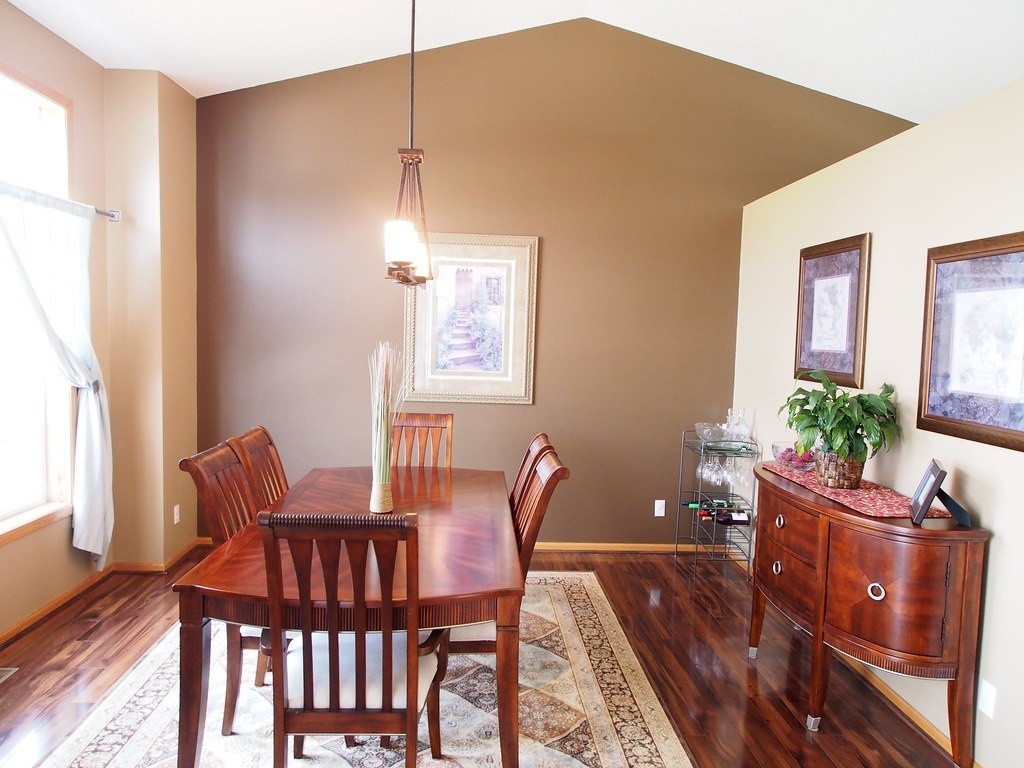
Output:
[907,458,972,528]
[916,230,1024,453]
[401,231,540,405]
[793,232,873,390]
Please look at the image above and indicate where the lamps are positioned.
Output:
[381,0,434,288]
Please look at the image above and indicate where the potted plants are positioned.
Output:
[776,369,904,490]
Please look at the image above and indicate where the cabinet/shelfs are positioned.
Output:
[746,460,992,768]
[673,429,759,580]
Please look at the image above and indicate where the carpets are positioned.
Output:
[34,570,700,768]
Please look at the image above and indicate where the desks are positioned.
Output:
[177,465,526,768]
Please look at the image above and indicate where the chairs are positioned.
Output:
[509,432,555,518]
[224,424,290,672]
[386,412,455,468]
[178,440,357,748]
[380,450,570,748]
[255,509,452,768]
